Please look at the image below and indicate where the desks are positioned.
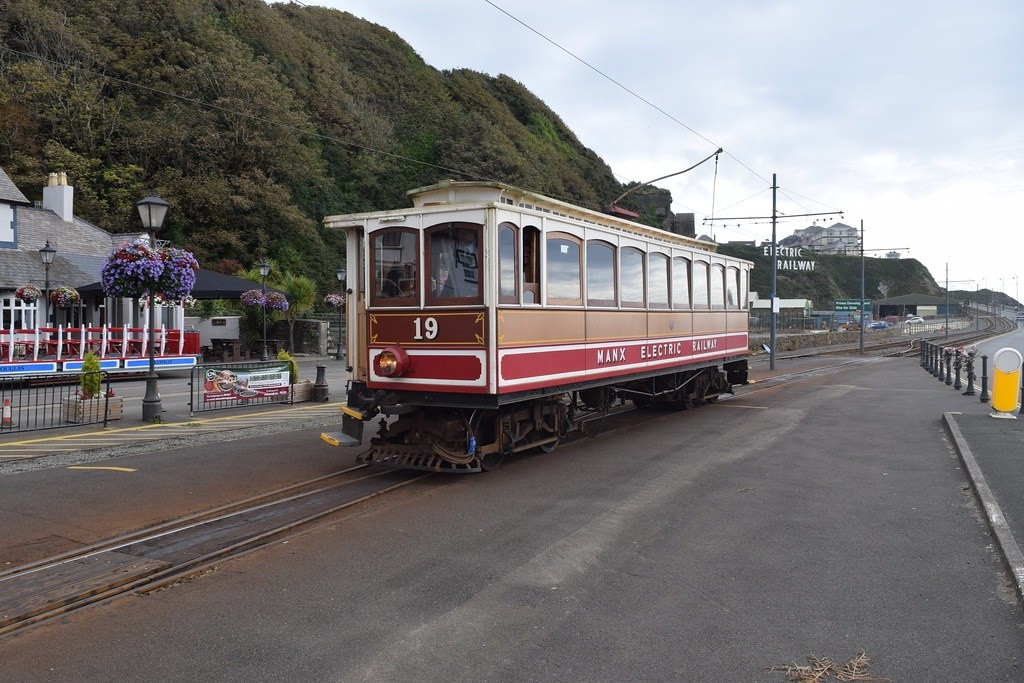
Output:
[257,339,285,353]
[209,338,241,360]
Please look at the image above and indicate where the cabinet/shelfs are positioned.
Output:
[0,323,186,363]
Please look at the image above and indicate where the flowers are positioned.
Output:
[940,347,955,367]
[240,290,267,308]
[961,347,983,382]
[14,284,42,304]
[324,292,343,308]
[267,293,289,312]
[951,346,966,367]
[50,286,81,308]
[101,243,199,307]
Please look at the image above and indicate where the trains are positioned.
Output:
[321,176,761,475]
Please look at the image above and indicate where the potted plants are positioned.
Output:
[62,350,123,423]
[277,349,316,404]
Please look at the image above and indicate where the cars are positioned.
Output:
[1016,310,1024,320]
[870,320,890,329]
[904,316,924,324]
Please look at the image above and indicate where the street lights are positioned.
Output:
[38,241,58,325]
[258,263,272,362]
[335,267,348,359]
[134,194,169,424]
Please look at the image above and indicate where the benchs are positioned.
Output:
[251,346,274,356]
[200,345,229,358]
[225,346,252,360]
